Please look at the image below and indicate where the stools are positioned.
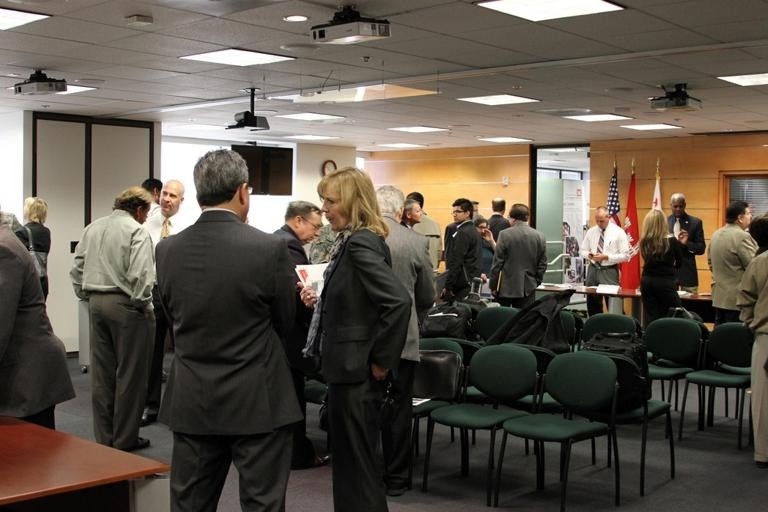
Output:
[304,380,328,405]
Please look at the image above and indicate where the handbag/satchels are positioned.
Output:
[23,226,48,278]
[584,332,652,416]
[470,278,484,298]
[412,349,465,400]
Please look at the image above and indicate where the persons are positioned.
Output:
[735,254,767,467]
[565,230,579,275]
[0,196,77,430]
[579,206,630,317]
[320,159,338,178]
[666,192,706,292]
[315,166,413,511]
[275,200,337,471]
[401,191,548,308]
[69,178,186,454]
[639,212,685,325]
[707,202,767,325]
[156,146,304,512]
[374,185,436,495]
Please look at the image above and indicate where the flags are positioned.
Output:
[618,173,640,290]
[651,177,662,211]
[606,171,621,226]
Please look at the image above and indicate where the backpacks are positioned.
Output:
[421,303,486,346]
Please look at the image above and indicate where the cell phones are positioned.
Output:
[589,254,593,257]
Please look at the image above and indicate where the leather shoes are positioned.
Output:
[124,437,150,451]
[314,454,331,466]
[138,407,158,426]
[387,481,415,496]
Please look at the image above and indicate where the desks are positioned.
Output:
[535,282,711,313]
[1,415,172,512]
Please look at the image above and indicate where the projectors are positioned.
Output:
[311,18,391,45]
[650,96,703,112]
[226,112,270,132]
[14,79,67,97]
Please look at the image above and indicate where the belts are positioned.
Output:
[592,263,615,270]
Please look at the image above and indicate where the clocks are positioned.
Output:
[322,159,337,176]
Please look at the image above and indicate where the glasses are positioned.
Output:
[301,216,320,230]
[451,210,464,215]
[245,181,254,194]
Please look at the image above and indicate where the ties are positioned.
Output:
[161,218,169,238]
[673,217,681,241]
[597,229,605,254]
